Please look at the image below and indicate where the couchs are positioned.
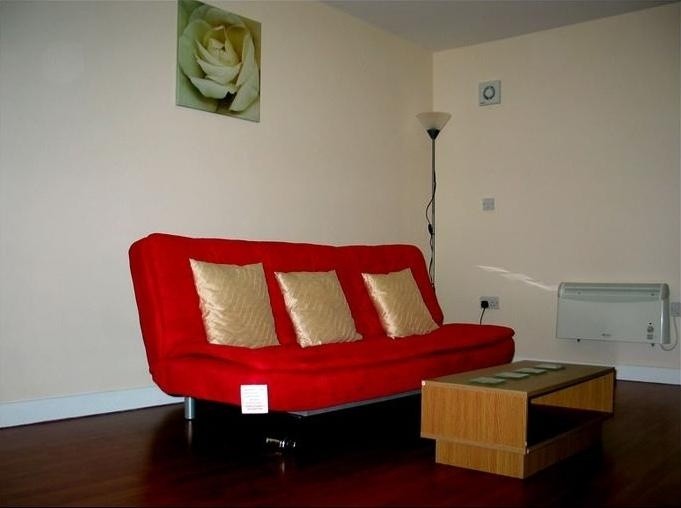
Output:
[129,233,515,474]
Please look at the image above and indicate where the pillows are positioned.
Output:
[189,257,280,349]
[361,267,440,339]
[274,269,363,348]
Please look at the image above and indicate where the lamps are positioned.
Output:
[415,111,453,292]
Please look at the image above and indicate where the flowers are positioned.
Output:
[178,3,261,113]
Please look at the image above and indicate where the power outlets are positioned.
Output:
[670,301,681,318]
[480,297,499,309]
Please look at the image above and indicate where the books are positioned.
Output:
[535,362,566,370]
[473,375,506,386]
[497,371,529,380]
[517,367,549,376]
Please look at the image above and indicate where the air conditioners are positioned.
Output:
[556,281,679,351]
